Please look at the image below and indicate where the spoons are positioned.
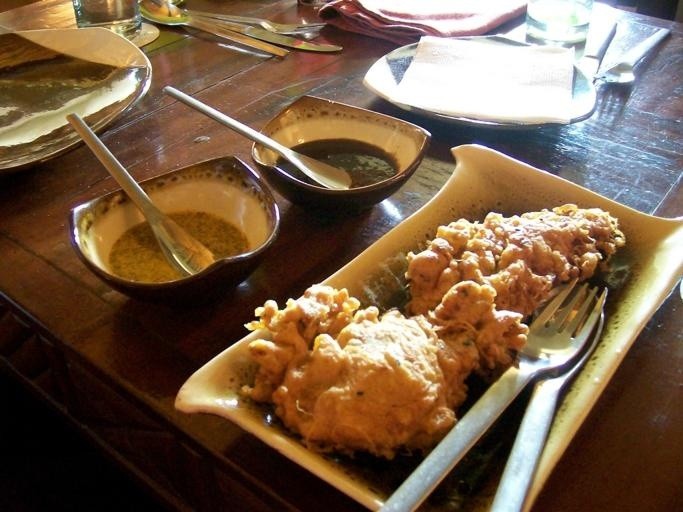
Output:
[65,113,220,278]
[160,83,351,191]
[137,0,290,57]
[485,282,605,511]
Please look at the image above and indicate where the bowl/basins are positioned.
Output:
[67,154,281,315]
[250,95,432,224]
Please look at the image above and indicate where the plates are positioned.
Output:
[172,141,683,511]
[0,28,153,171]
[363,34,597,132]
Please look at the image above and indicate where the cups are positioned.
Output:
[526,1,593,52]
[71,0,141,41]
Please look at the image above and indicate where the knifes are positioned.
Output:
[193,15,344,52]
[579,22,617,84]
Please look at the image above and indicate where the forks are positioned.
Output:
[593,28,673,87]
[370,277,609,511]
[181,7,328,35]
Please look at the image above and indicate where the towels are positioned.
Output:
[318,0,528,53]
